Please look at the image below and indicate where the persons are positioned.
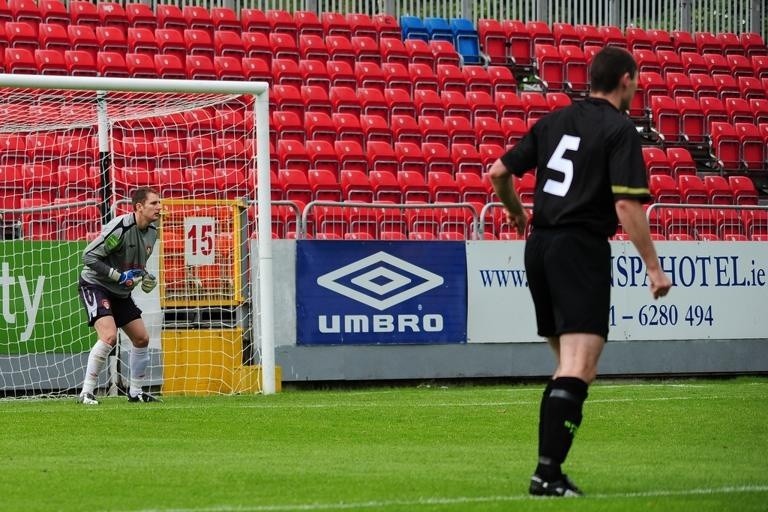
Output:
[485,46,673,499]
[74,185,163,406]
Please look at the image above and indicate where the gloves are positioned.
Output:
[116,269,142,289]
[140,274,157,293]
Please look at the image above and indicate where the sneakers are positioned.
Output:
[529,474,582,497]
[77,394,101,405]
[128,392,161,402]
